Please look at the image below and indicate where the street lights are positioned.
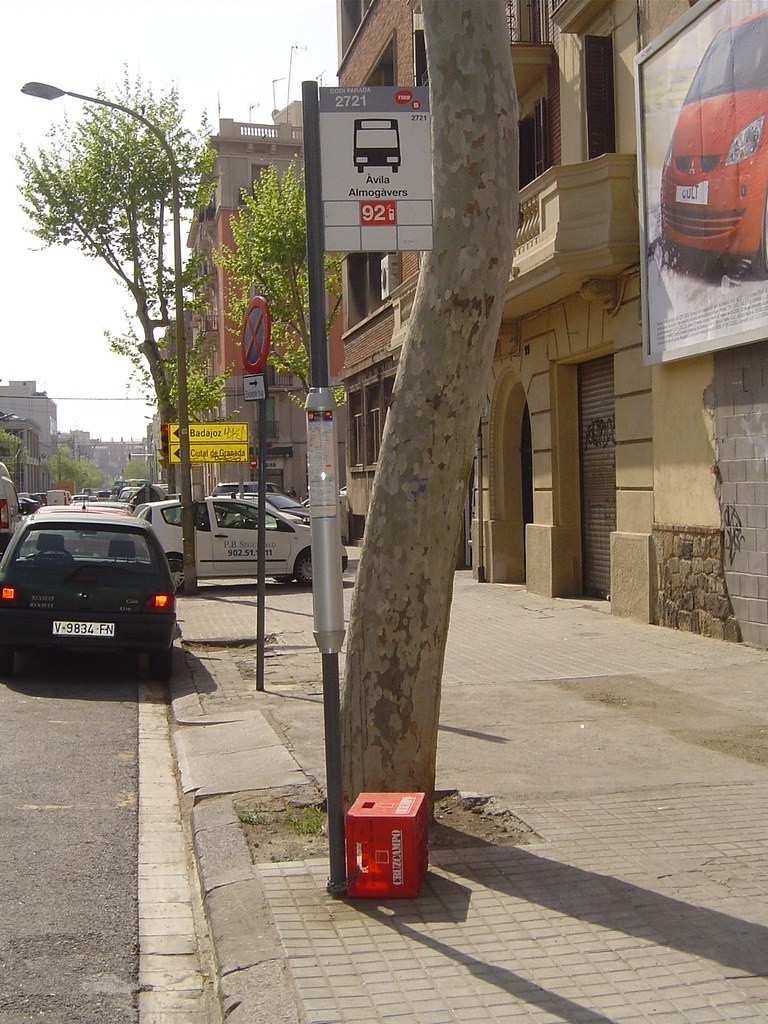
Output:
[22,80,199,599]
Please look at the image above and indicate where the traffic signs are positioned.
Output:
[168,442,249,463]
[167,421,249,444]
[243,373,265,402]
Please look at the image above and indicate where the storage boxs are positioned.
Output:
[346,793,428,898]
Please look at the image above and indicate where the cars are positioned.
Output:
[131,491,348,596]
[660,6,768,280]
[0,514,183,682]
[236,491,310,525]
[16,479,181,556]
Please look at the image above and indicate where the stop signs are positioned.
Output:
[250,459,258,468]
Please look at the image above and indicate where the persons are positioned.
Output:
[287,486,297,498]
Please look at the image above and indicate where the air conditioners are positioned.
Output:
[380,255,403,302]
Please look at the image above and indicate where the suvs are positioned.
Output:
[211,481,286,498]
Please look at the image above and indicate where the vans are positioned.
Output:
[0,462,29,556]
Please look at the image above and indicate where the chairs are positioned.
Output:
[36,534,64,559]
[166,508,177,524]
[108,540,135,563]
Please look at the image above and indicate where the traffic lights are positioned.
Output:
[157,448,167,469]
[160,424,168,453]
